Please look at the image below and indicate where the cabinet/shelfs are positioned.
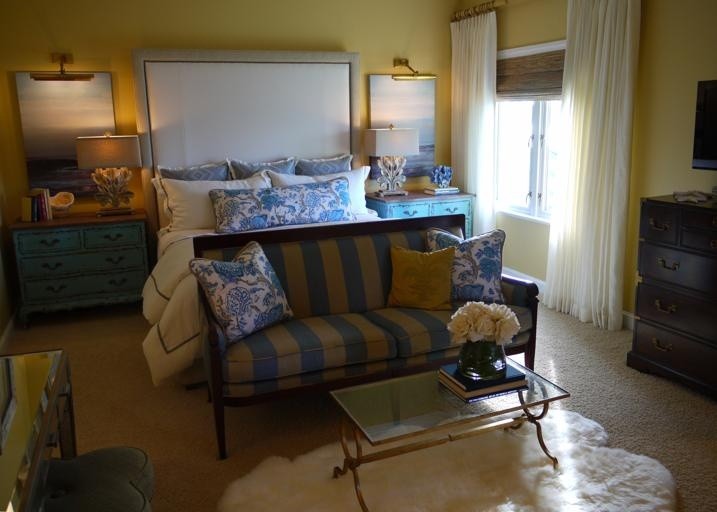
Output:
[625,191,717,403]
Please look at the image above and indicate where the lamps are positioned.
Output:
[392,57,437,81]
[362,123,420,198]
[75,134,142,218]
[30,51,94,83]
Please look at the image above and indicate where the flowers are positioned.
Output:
[447,301,520,346]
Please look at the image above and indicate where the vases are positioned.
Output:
[456,341,507,382]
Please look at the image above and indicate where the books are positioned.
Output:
[437,359,530,404]
[423,186,460,196]
[18,187,54,222]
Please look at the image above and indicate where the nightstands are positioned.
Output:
[365,190,473,240]
[9,209,149,328]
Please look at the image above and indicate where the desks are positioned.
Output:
[0,349,77,512]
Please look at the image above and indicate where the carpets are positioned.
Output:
[220,410,677,511]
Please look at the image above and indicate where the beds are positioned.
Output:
[142,152,387,395]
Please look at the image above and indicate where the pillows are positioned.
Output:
[189,242,294,345]
[226,157,296,180]
[386,245,456,311]
[209,176,358,234]
[295,154,353,176]
[425,228,507,304]
[151,171,273,232]
[156,161,227,181]
[267,166,370,215]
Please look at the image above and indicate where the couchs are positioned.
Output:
[192,213,540,458]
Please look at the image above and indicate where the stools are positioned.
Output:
[41,445,153,512]
